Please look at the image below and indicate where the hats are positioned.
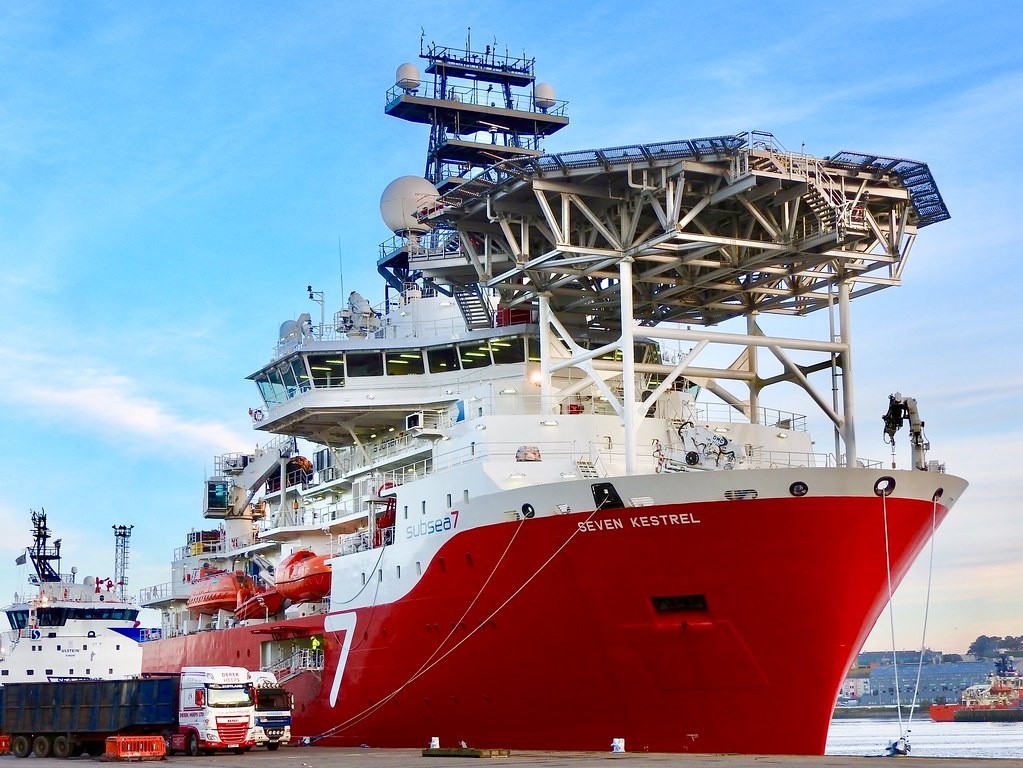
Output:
[310,636,315,641]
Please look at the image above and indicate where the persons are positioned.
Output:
[311,636,321,667]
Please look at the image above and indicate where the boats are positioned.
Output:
[141,20,971,758]
[0,506,162,683]
[928,648,1023,723]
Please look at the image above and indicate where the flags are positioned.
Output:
[15,553,26,566]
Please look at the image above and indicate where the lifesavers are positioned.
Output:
[254,410,264,421]
[851,206,863,222]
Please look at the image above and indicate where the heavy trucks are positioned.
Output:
[0,666,295,758]
